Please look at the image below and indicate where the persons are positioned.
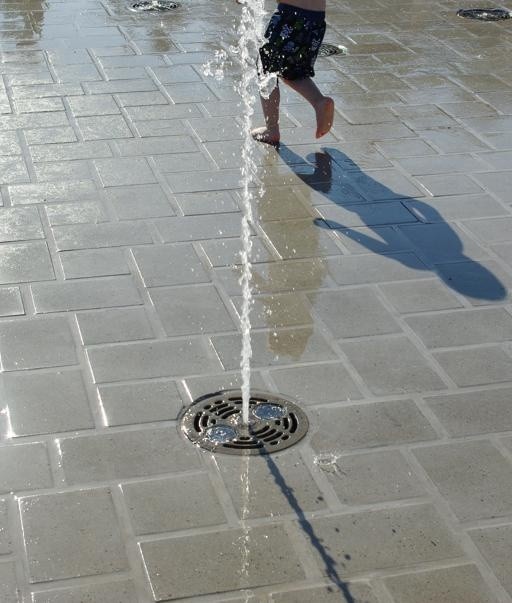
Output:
[250,0,336,145]
